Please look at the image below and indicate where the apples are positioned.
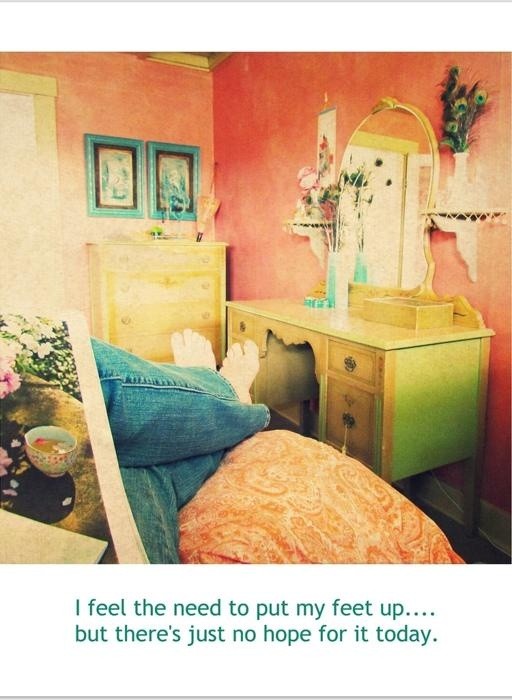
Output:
[150,226,162,235]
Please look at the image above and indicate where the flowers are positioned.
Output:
[341,166,378,256]
[287,161,349,269]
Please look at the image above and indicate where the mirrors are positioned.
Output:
[340,146,451,329]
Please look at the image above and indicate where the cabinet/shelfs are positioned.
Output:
[87,238,227,371]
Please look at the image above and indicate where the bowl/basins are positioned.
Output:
[22,424,78,479]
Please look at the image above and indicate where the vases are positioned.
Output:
[327,251,351,312]
[352,248,370,286]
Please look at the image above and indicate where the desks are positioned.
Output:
[225,298,498,540]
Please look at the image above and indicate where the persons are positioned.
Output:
[82,327,271,564]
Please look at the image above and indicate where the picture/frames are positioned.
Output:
[81,131,200,225]
